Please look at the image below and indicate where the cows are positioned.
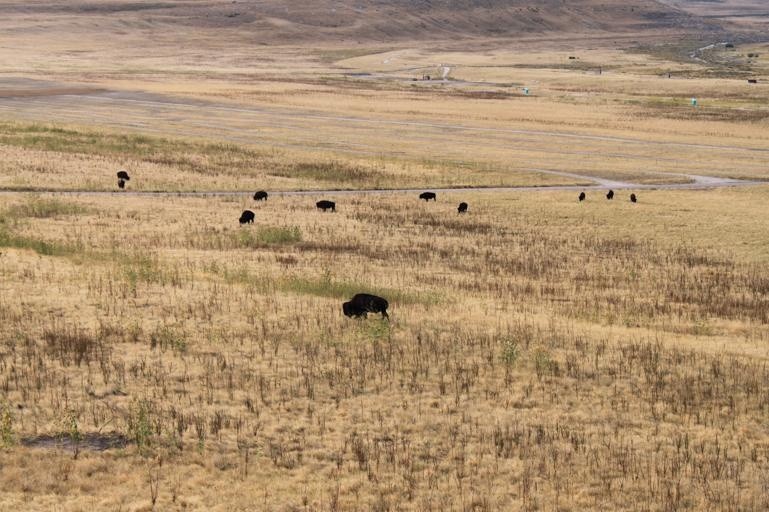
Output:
[117,171,130,189]
[607,190,614,199]
[631,194,637,202]
[458,202,467,213]
[343,293,389,320]
[239,210,255,224]
[316,200,335,212]
[253,191,268,200]
[579,192,585,201]
[420,192,436,201]
